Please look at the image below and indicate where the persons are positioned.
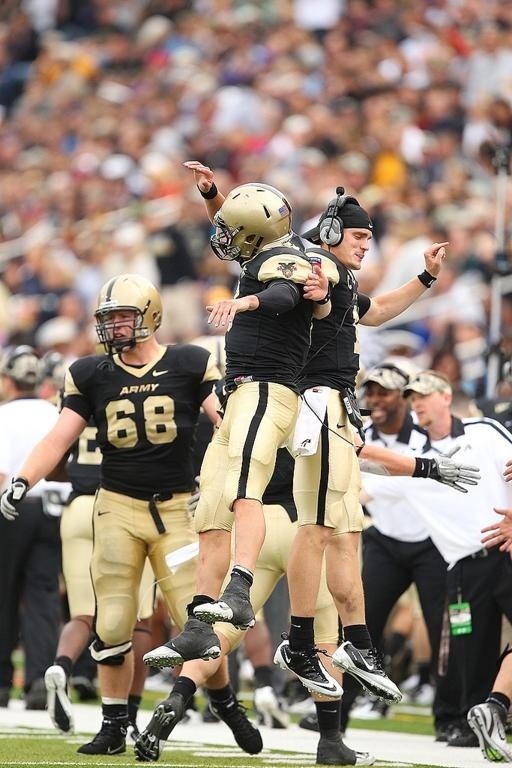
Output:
[1,1,512,768]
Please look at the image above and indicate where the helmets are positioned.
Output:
[2,344,44,386]
[210,183,292,267]
[95,274,162,356]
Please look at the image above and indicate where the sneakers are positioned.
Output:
[44,663,74,736]
[142,619,221,669]
[135,693,186,761]
[193,590,255,631]
[26,680,45,711]
[78,720,126,755]
[72,676,98,700]
[466,700,511,762]
[254,686,290,728]
[209,693,263,754]
[300,713,346,736]
[436,719,478,746]
[0,687,9,707]
[318,736,376,767]
[331,640,403,706]
[274,632,345,700]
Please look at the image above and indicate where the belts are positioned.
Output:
[468,544,507,560]
[100,483,196,536]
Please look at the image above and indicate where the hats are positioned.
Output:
[361,364,408,390]
[301,203,372,244]
[401,371,453,398]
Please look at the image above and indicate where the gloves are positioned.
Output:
[0,478,30,521]
[412,445,481,493]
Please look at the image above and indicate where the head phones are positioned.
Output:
[320,195,362,247]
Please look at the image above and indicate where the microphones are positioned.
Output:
[326,185,344,237]
